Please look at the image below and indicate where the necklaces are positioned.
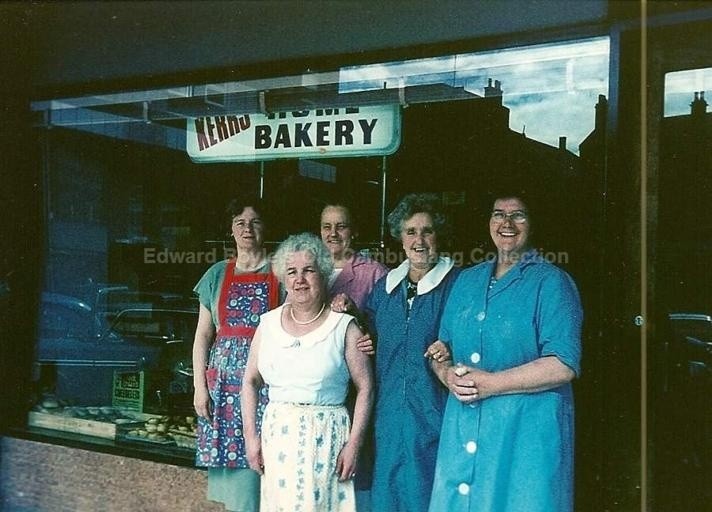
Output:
[289,301,326,325]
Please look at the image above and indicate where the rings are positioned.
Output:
[350,470,357,477]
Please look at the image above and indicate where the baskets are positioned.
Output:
[168,429,198,450]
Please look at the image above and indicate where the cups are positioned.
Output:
[75,406,169,442]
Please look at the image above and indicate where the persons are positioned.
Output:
[191,195,288,510]
[328,195,461,512]
[239,233,374,512]
[318,203,393,494]
[426,181,582,511]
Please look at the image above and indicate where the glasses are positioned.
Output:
[491,211,528,224]
